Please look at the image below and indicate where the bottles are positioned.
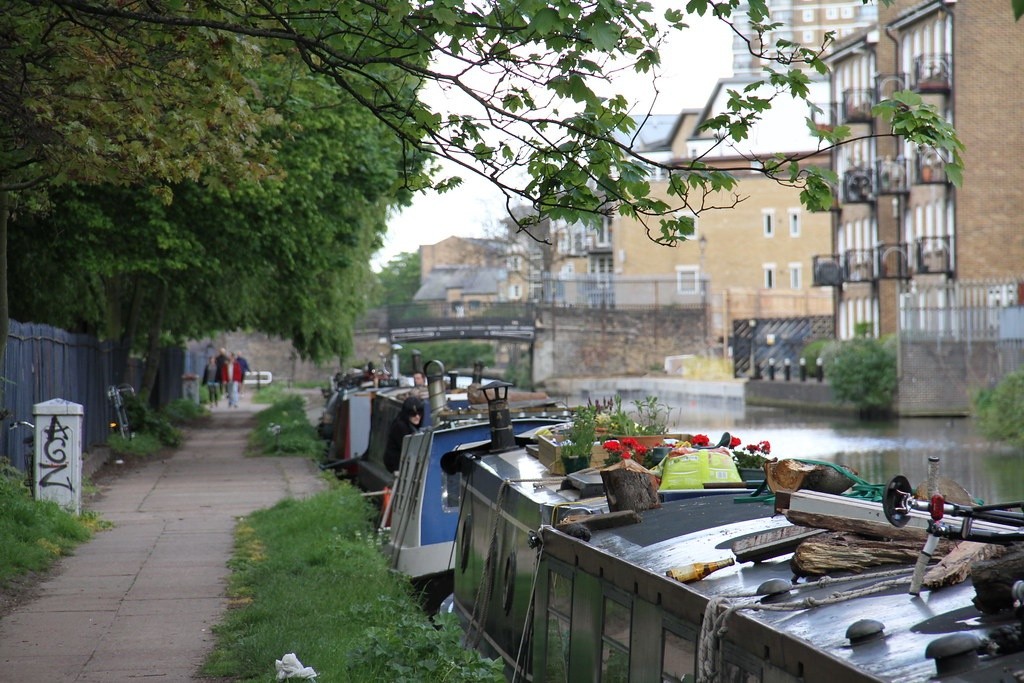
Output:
[666,558,735,585]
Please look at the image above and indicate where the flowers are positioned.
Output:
[603,436,647,466]
[653,441,675,448]
[690,434,711,450]
[730,436,770,467]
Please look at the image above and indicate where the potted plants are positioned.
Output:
[730,436,771,481]
[609,394,676,451]
[554,403,595,475]
[585,395,613,438]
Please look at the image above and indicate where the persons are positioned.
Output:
[215,348,229,395]
[236,351,252,393]
[413,371,425,387]
[201,356,222,408]
[221,351,242,409]
[384,396,424,473]
